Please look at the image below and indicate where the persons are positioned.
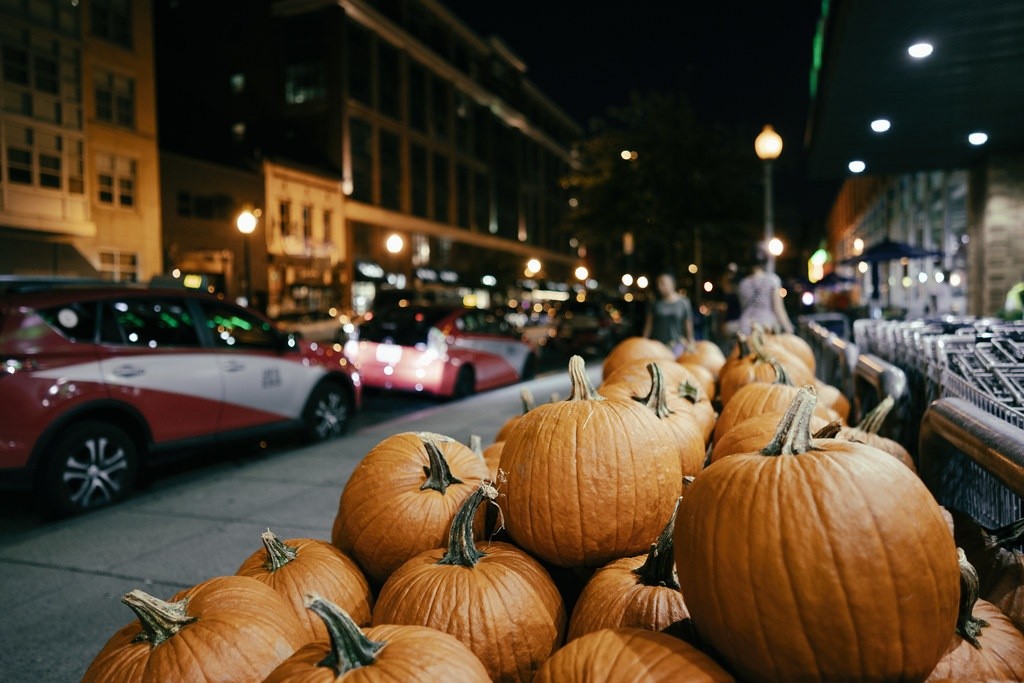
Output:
[735,241,797,348]
[643,270,694,351]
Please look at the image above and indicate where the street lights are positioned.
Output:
[754,123,783,275]
[237,209,258,309]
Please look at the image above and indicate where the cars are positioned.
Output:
[342,302,540,401]
[494,292,648,361]
[0,283,363,519]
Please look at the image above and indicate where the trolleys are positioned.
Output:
[850,312,1024,550]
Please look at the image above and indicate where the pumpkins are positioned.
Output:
[83,333,1024,683]
